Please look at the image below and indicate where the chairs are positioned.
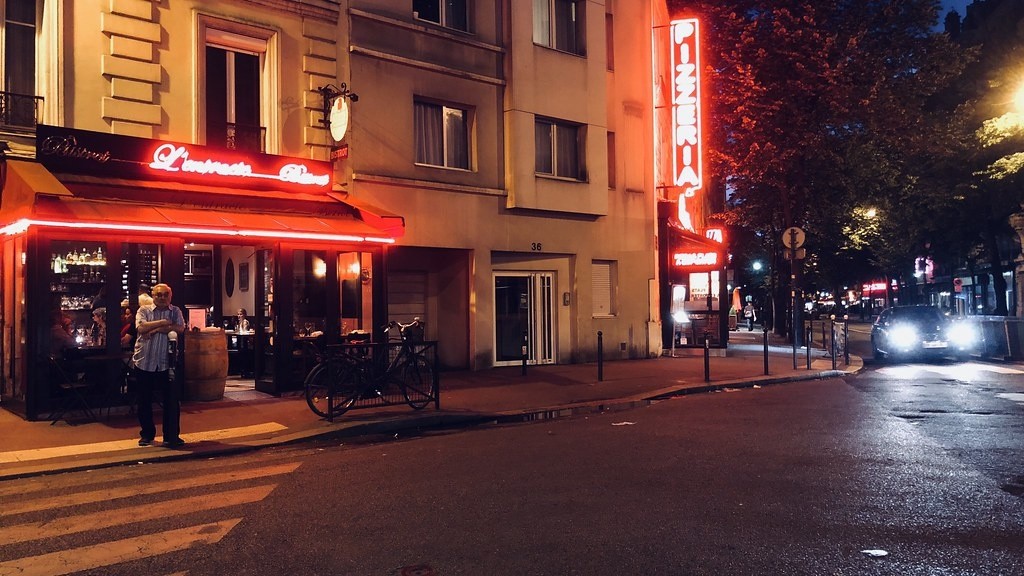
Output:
[128,341,176,419]
[44,351,98,426]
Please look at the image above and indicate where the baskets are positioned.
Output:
[398,322,425,342]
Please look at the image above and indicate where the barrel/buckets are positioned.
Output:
[184,331,228,401]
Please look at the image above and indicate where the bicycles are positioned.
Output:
[305,316,433,417]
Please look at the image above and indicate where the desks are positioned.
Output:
[84,352,135,421]
[225,330,270,375]
[688,317,720,346]
[293,332,371,358]
[75,341,99,347]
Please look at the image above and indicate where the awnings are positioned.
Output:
[0,160,405,238]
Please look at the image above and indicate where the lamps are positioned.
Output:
[318,82,358,142]
[51,247,106,274]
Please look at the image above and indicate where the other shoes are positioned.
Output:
[163,438,184,446]
[139,438,154,445]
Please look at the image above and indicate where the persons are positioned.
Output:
[132,283,186,446]
[50,287,133,349]
[744,302,756,331]
[235,308,250,331]
[137,284,154,306]
[941,301,948,311]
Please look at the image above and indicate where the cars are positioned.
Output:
[803,297,819,318]
[871,307,972,364]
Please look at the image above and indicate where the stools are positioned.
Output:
[682,330,693,345]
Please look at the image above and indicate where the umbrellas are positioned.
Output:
[732,288,742,313]
